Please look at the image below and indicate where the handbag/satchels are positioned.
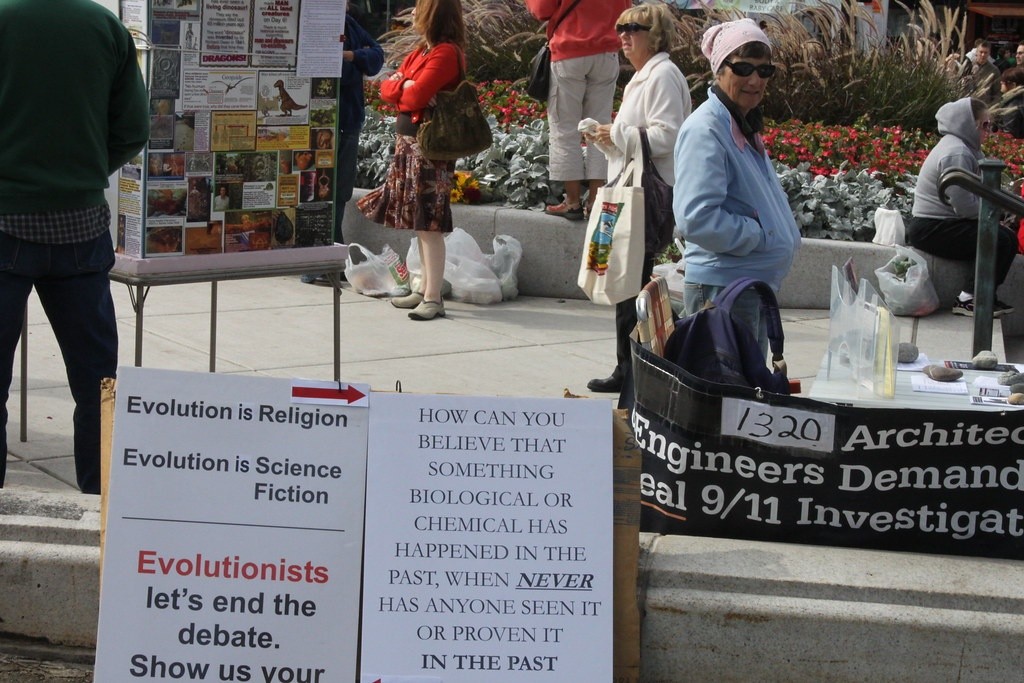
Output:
[874,244,941,317]
[527,46,551,105]
[872,207,905,247]
[343,226,523,304]
[416,38,492,161]
[639,126,674,257]
[577,127,645,306]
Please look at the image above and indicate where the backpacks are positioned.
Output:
[663,277,790,396]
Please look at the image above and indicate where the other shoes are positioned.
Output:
[586,375,621,393]
[408,297,445,320]
[390,291,424,309]
[582,200,594,219]
[545,200,584,221]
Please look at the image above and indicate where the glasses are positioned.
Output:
[977,120,991,131]
[723,59,776,78]
[617,22,652,36]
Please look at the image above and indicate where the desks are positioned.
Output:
[809,351,1024,412]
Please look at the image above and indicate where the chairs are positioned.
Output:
[636,276,802,395]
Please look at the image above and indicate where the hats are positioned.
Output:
[701,18,773,74]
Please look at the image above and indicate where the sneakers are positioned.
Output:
[995,299,1015,314]
[952,296,1005,317]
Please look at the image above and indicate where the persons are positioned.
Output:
[301,1,385,286]
[525,0,632,220]
[214,187,230,211]
[908,98,1019,319]
[952,37,1024,140]
[672,19,800,368]
[356,0,468,320]
[162,156,179,177]
[583,4,692,392]
[0,0,151,496]
[217,154,245,174]
